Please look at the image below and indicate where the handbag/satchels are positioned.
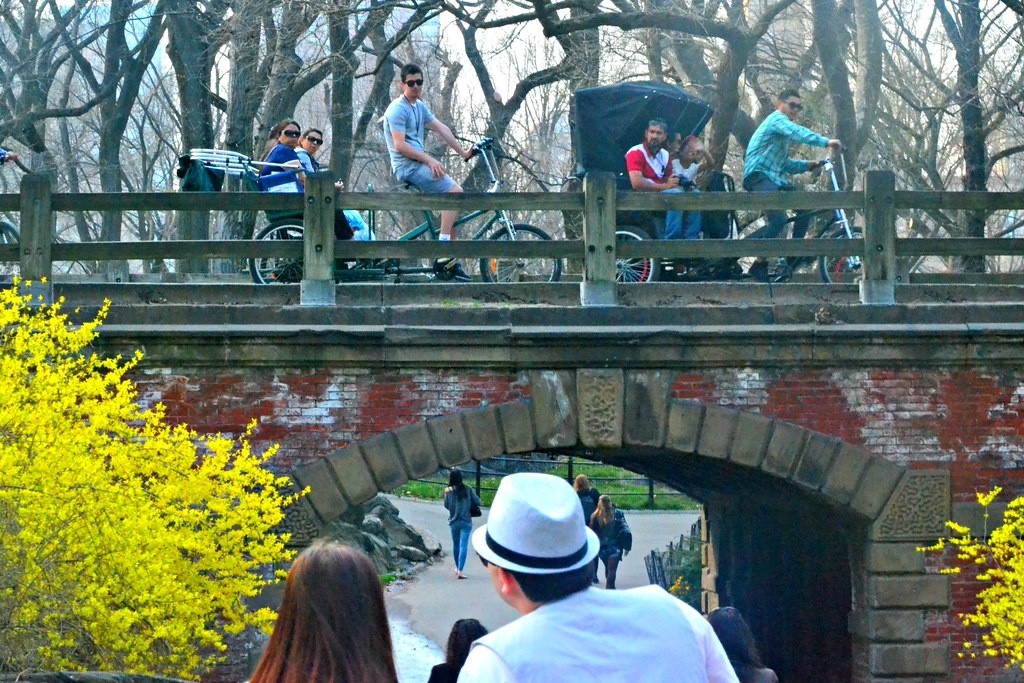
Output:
[176,153,224,193]
[617,525,633,552]
[469,486,481,517]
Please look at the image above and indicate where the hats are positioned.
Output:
[679,135,698,151]
[471,471,600,575]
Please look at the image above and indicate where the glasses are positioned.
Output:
[281,129,301,139]
[304,135,324,146]
[782,99,804,112]
[403,79,424,88]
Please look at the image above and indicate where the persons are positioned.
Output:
[426,618,489,683]
[249,535,404,683]
[258,119,355,241]
[705,606,779,683]
[455,472,741,683]
[574,474,601,583]
[623,116,701,266]
[590,495,629,589]
[670,131,715,191]
[294,126,388,265]
[742,88,843,283]
[384,62,474,281]
[444,467,480,579]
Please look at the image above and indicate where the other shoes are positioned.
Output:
[789,254,817,268]
[747,259,781,283]
[452,567,459,573]
[457,574,467,579]
[659,256,687,275]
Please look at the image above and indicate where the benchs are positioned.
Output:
[243,164,306,221]
[610,172,725,228]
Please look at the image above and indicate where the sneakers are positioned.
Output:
[432,258,473,281]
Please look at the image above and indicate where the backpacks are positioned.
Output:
[576,487,595,525]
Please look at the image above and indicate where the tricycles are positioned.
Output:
[0,152,31,244]
[177,135,562,284]
[564,79,864,283]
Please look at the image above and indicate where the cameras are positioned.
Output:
[675,174,694,191]
